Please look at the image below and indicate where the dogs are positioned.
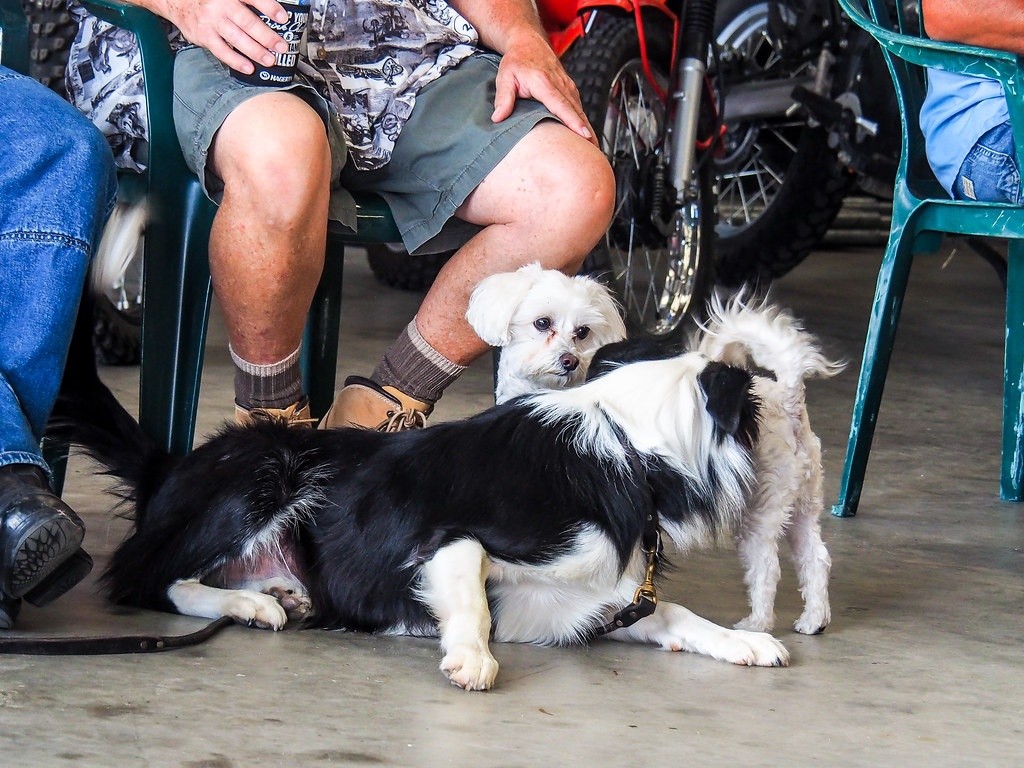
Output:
[44,259,852,693]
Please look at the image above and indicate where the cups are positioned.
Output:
[230,0,311,87]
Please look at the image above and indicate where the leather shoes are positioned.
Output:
[0,591,22,629]
[0,462,94,608]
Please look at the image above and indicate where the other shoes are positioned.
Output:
[233,393,319,429]
[315,374,434,432]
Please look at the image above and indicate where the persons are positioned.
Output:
[919,0,1024,203]
[0,64,118,629]
[65,0,616,432]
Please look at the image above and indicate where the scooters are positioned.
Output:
[513,1,918,362]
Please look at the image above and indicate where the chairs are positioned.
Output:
[1,0,506,500]
[832,0,1024,520]
